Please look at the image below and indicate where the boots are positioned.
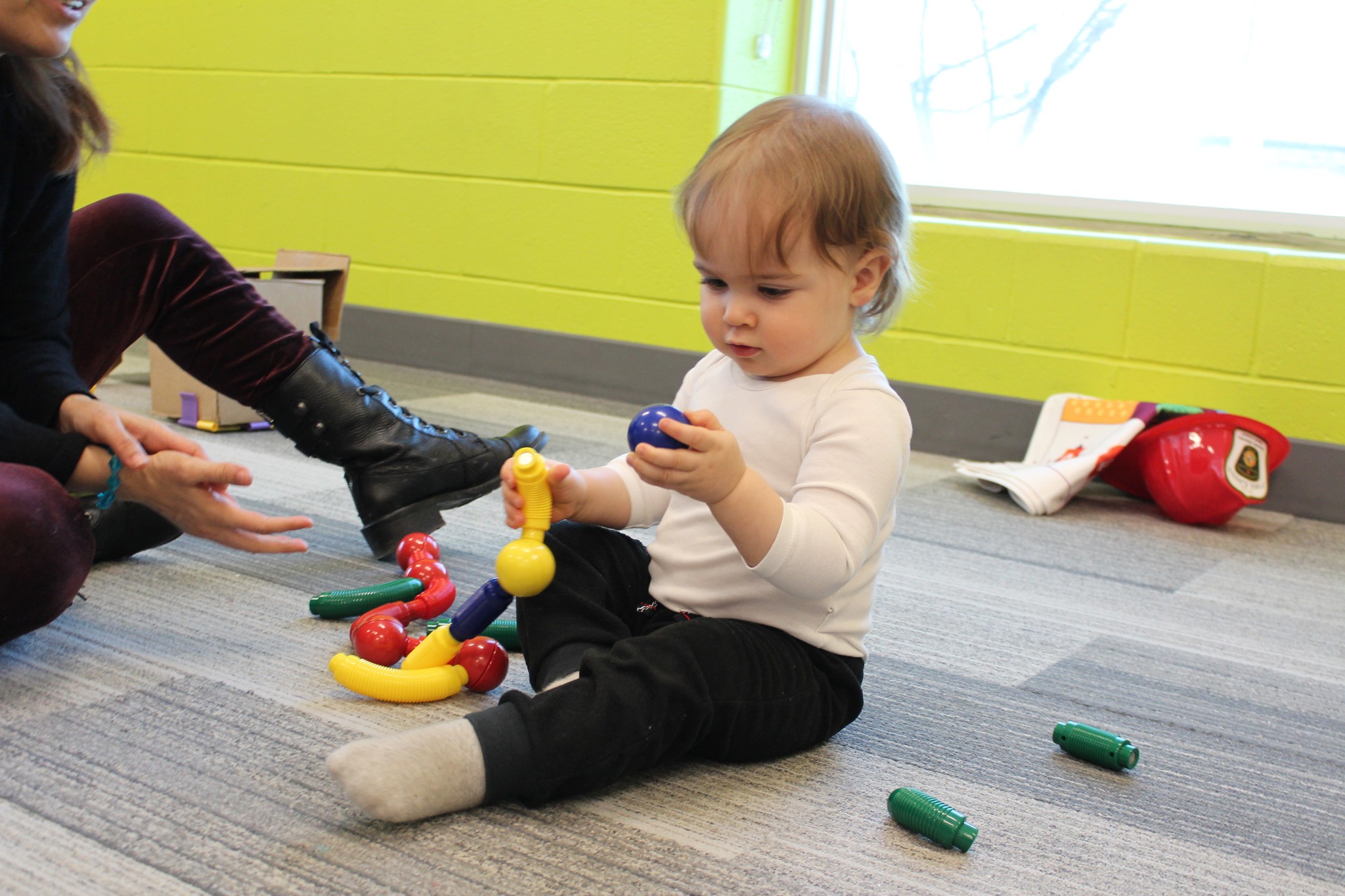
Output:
[257,323,544,559]
[85,496,182,563]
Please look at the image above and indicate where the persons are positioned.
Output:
[327,96,913,823]
[0,0,546,646]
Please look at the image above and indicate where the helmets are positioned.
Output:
[1098,411,1292,526]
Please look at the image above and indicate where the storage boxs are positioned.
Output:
[146,250,351,429]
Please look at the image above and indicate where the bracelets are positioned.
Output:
[83,454,123,530]
